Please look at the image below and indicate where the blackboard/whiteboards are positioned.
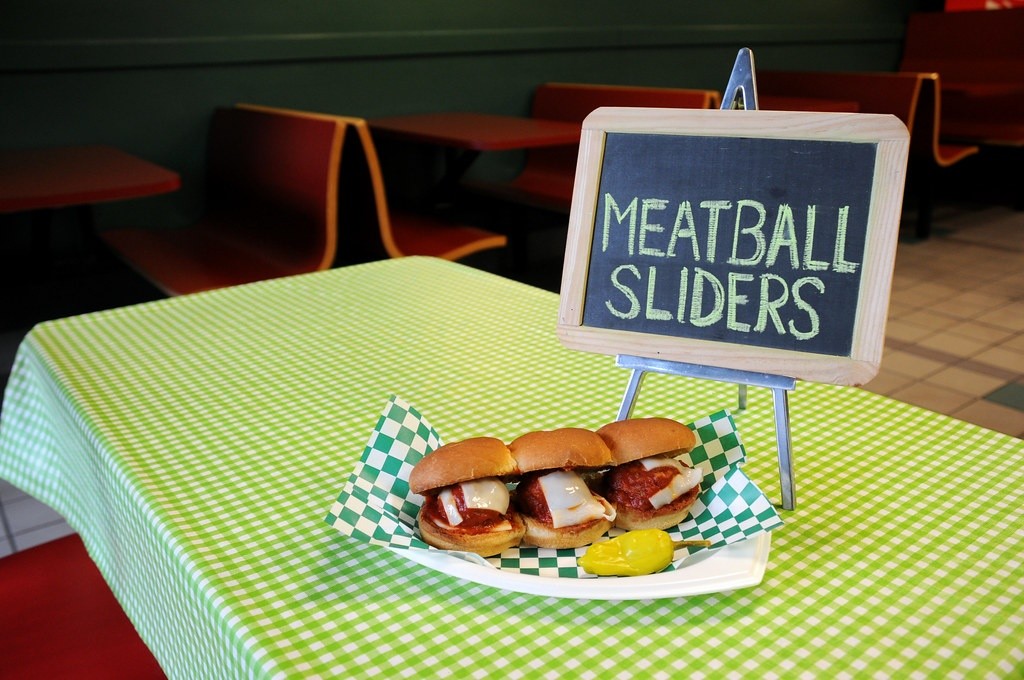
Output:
[557,107,911,388]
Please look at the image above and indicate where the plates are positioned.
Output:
[382,531,772,601]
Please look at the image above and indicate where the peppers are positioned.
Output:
[576,528,712,576]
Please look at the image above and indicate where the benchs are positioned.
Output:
[762,69,924,137]
[507,83,713,215]
[104,104,346,297]
[914,72,980,239]
[343,118,507,274]
[901,13,1024,210]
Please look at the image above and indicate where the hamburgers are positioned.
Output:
[409,416,704,557]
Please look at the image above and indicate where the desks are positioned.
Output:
[946,78,1024,96]
[0,145,181,313]
[758,94,860,113]
[34,253,1024,680]
[368,112,582,221]
